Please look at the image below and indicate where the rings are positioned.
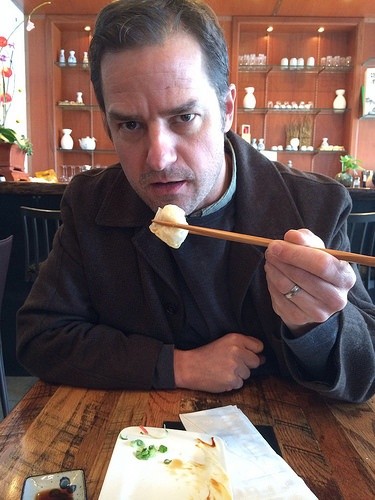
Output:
[282,284,303,299]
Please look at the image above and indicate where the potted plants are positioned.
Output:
[335,155,365,186]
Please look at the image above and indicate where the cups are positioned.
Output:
[363,170,373,186]
[321,56,352,72]
[280,57,315,70]
[61,165,107,182]
[239,53,266,70]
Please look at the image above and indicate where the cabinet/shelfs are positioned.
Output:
[54,15,368,183]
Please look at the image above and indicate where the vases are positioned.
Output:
[61,129,74,150]
[0,144,30,182]
[333,89,346,113]
[242,86,255,110]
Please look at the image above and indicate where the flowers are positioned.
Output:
[0,1,52,154]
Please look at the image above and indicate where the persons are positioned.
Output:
[16,0,375,404]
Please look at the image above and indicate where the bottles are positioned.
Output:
[76,92,83,103]
[251,138,265,151]
[353,177,360,188]
[60,128,73,149]
[320,138,328,147]
[333,89,346,113]
[68,50,77,66]
[84,52,88,67]
[59,49,64,67]
[243,86,256,112]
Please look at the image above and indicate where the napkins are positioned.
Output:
[179,405,320,500]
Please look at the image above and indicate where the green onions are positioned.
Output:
[121,434,172,463]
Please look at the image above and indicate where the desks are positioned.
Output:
[0,373,375,500]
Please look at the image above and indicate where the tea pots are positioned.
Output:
[78,136,96,150]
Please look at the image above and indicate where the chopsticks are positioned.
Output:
[152,219,375,269]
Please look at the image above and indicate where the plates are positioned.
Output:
[20,468,87,500]
[97,427,231,500]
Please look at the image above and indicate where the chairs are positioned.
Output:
[0,235,14,417]
[21,206,64,302]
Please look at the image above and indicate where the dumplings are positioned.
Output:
[150,204,190,248]
